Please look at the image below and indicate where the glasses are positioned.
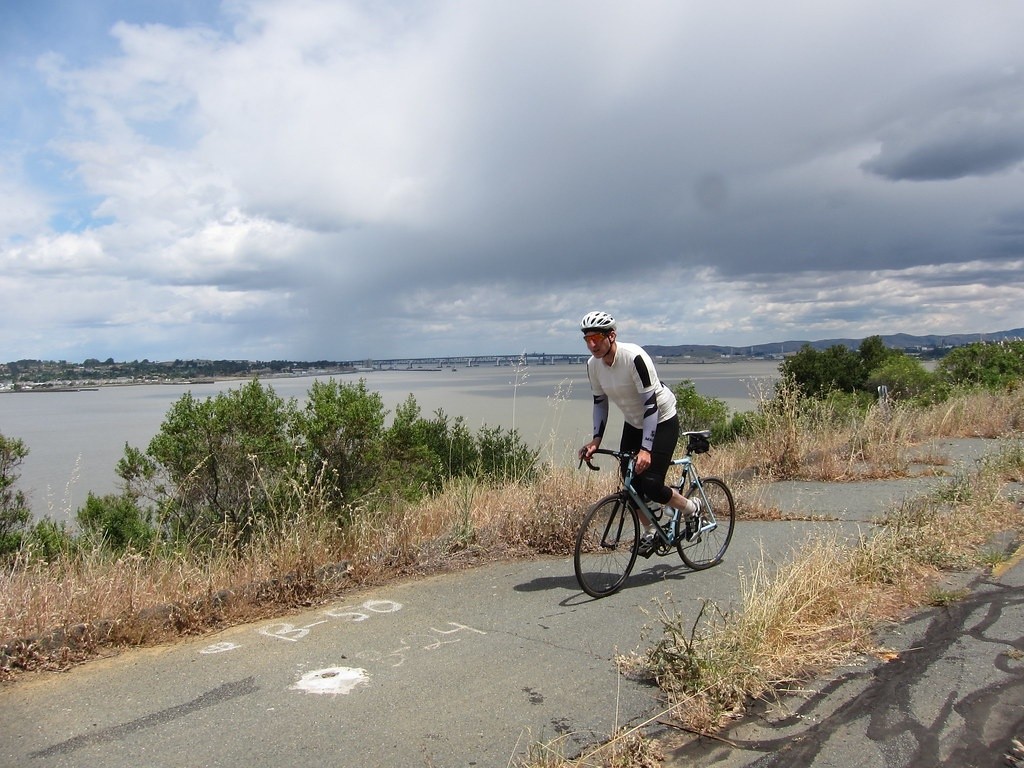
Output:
[583,333,609,342]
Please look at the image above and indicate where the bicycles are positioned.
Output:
[574,431,736,599]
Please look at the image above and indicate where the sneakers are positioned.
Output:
[629,531,663,553]
[685,497,705,542]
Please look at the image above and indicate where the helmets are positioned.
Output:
[580,311,617,336]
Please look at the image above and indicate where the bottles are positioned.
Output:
[664,480,680,518]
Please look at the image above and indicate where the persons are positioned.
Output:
[579,311,703,554]
